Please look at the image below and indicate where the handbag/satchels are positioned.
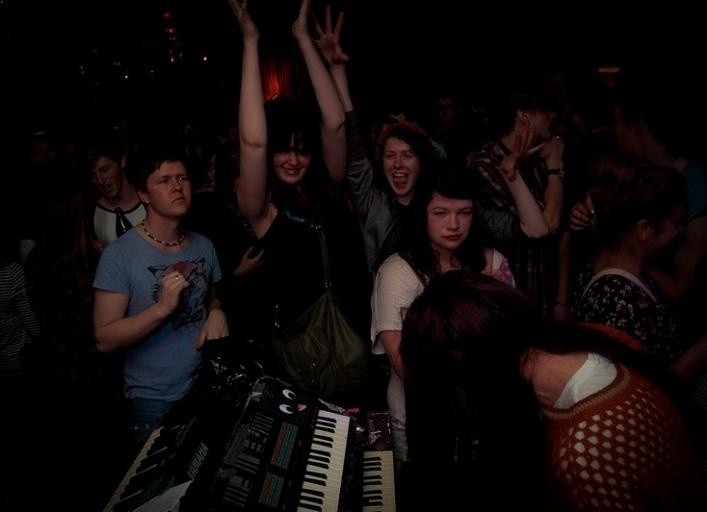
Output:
[272,292,366,399]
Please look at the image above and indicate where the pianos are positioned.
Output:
[103,375,399,512]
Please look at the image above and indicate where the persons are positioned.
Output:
[1,4,707,511]
